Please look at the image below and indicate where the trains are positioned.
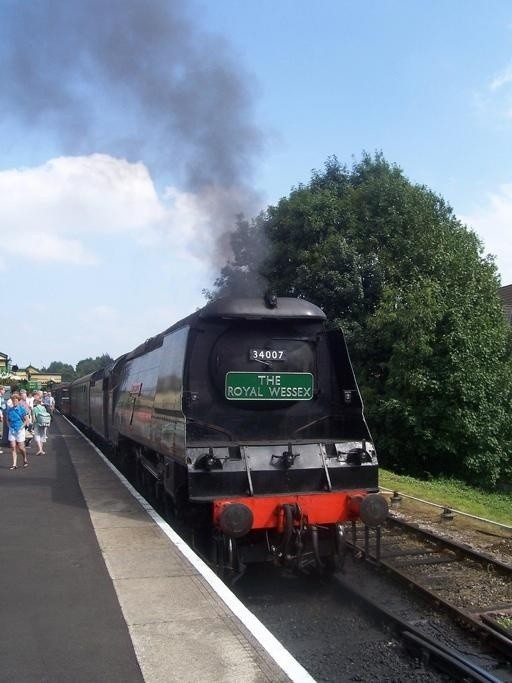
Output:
[40,285,389,585]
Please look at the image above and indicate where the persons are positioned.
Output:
[0,384,56,470]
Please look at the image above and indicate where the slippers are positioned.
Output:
[10,464,19,470]
[24,460,30,467]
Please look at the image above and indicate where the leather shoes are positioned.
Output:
[36,451,43,456]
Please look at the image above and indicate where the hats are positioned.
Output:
[19,389,51,395]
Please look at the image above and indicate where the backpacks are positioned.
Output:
[36,405,51,427]
